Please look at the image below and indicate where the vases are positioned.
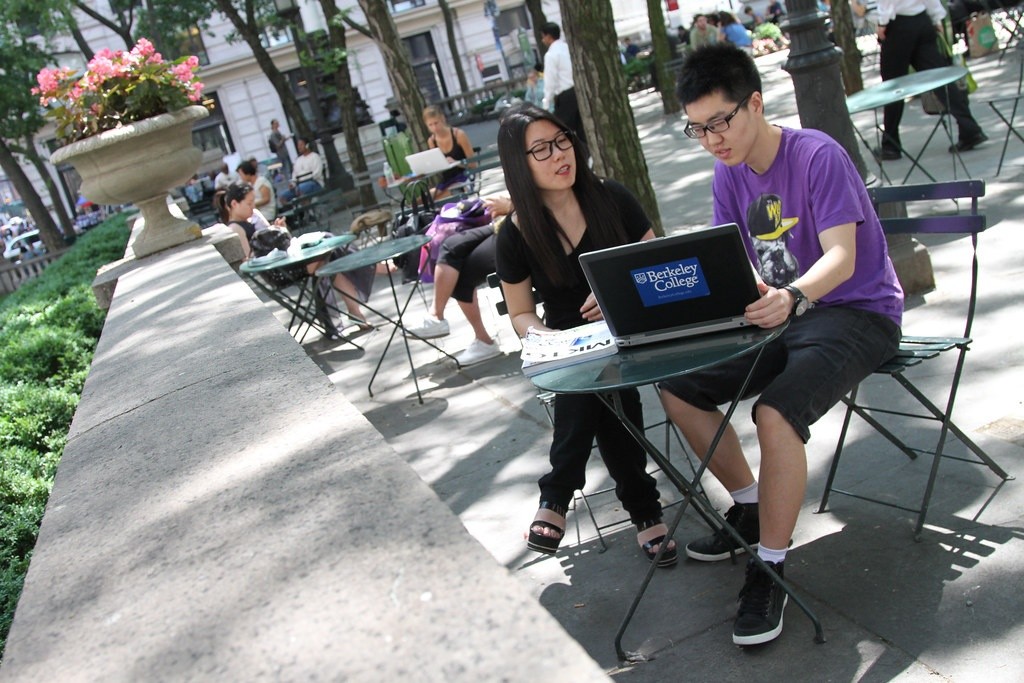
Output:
[50,105,211,259]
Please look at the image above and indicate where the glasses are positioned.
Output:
[525,131,575,161]
[683,92,749,139]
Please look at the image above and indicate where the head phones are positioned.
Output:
[300,137,311,150]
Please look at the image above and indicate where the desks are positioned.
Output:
[846,64,973,216]
[314,235,461,405]
[383,160,461,212]
[238,234,379,352]
[520,315,826,669]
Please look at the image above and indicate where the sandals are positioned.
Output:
[526,499,568,556]
[635,518,679,566]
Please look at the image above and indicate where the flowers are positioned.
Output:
[30,38,205,144]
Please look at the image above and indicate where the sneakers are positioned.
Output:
[732,558,788,645]
[399,315,450,340]
[685,500,761,561]
[451,337,500,366]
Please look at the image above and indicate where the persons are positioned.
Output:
[213,182,398,330]
[523,22,591,161]
[621,0,784,57]
[410,104,476,207]
[657,42,905,647]
[398,198,514,366]
[492,107,676,566]
[872,0,989,160]
[189,119,325,225]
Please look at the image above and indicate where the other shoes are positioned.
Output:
[347,314,370,329]
[374,261,398,275]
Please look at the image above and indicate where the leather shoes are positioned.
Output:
[949,129,988,154]
[873,144,901,160]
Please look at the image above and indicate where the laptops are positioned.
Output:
[577,223,763,347]
[404,147,460,176]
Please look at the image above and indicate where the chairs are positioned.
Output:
[268,147,550,328]
[974,0,1024,69]
[976,55,1024,178]
[812,177,1014,541]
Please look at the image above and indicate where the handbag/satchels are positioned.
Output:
[417,199,490,283]
[922,32,969,113]
[390,179,443,285]
[936,32,979,93]
[964,9,1003,57]
[247,224,292,258]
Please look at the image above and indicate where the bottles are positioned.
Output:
[383,163,395,185]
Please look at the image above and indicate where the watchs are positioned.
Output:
[785,284,809,319]
